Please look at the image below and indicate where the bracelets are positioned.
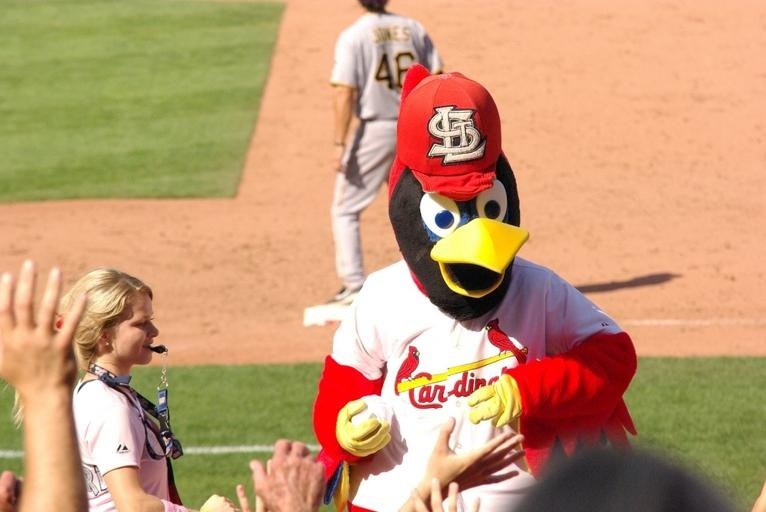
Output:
[331,141,348,149]
[159,498,196,512]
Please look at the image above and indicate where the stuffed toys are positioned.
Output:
[308,59,640,512]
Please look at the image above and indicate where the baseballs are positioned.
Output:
[351,396,393,428]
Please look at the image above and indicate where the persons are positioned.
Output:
[50,267,197,512]
[326,0,445,302]
[1,433,766,512]
[0,258,91,512]
